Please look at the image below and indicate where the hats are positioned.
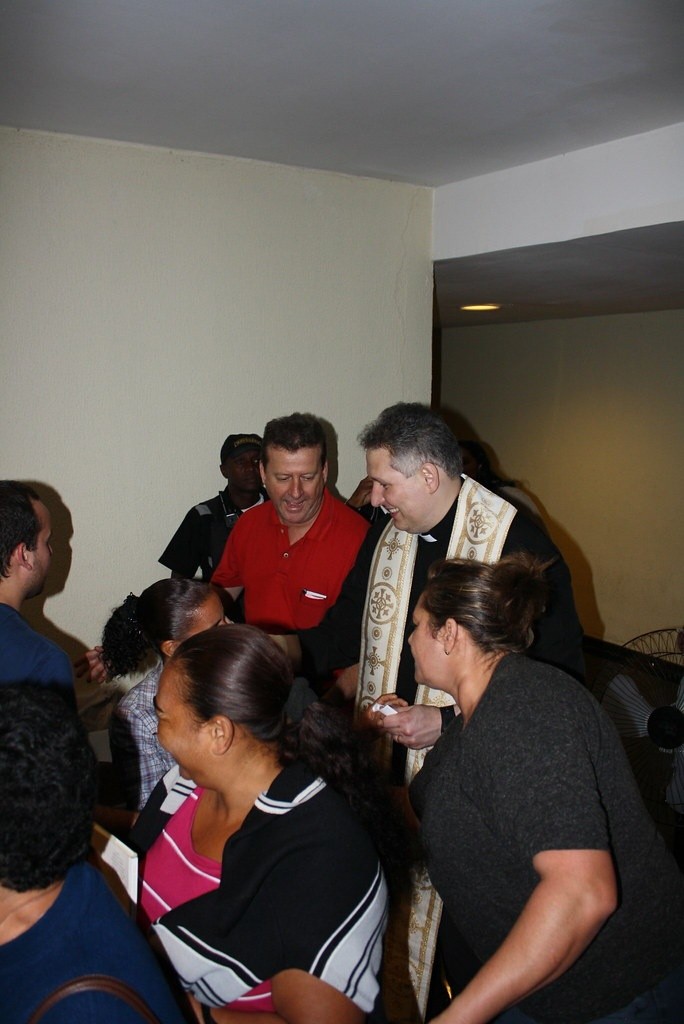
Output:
[221,434,265,464]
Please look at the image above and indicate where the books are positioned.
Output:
[88,822,139,922]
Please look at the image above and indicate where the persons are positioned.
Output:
[0,403,684,1024]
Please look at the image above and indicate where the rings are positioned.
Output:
[397,735,400,742]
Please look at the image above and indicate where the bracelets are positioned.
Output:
[440,706,456,734]
